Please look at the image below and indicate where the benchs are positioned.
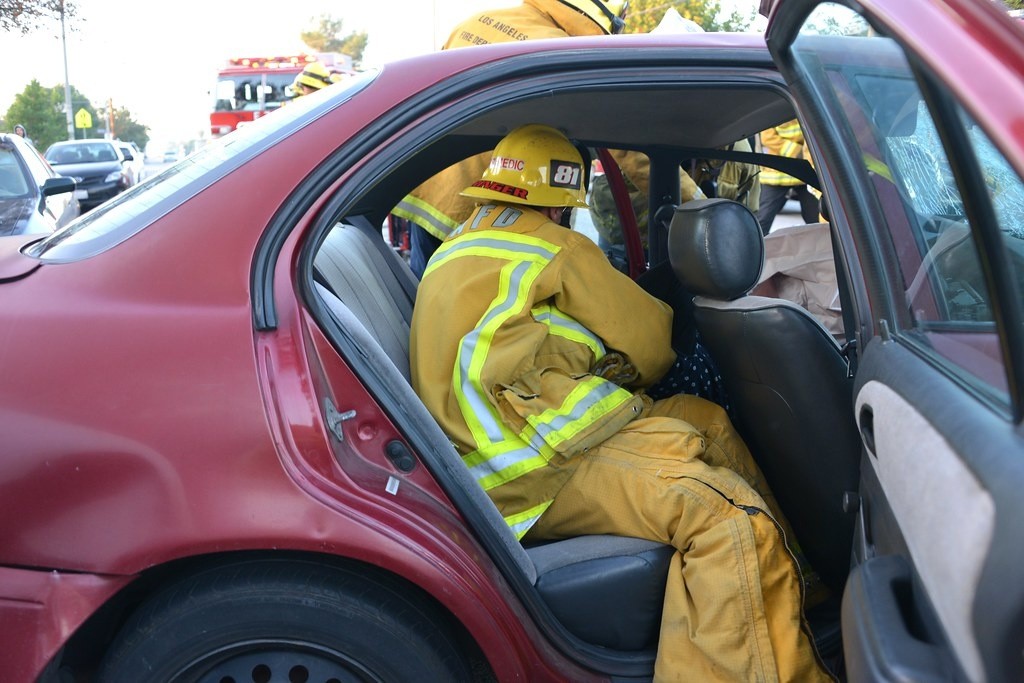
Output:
[312,215,678,651]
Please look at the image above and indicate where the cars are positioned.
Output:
[0,132,79,258]
[44,139,146,211]
[0,1,1023,682]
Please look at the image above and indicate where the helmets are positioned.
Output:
[459,123,591,209]
[559,0,630,35]
[298,63,334,89]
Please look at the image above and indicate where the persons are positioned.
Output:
[759,107,831,236]
[281,62,346,107]
[390,1,630,282]
[591,130,760,278]
[14,125,34,147]
[411,122,839,683]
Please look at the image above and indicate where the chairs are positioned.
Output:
[97,151,114,160]
[0,168,17,193]
[667,198,862,588]
[62,151,79,163]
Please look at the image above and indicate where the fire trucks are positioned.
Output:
[209,51,352,136]
